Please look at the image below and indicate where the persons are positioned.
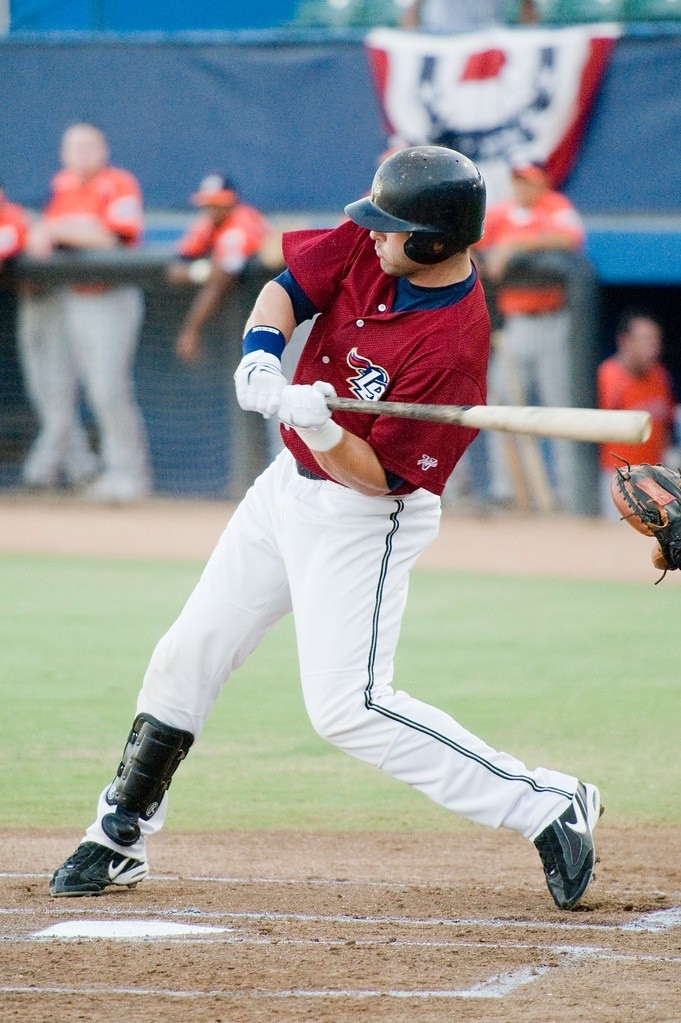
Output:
[50,146,601,910]
[598,315,681,521]
[171,174,281,363]
[0,121,151,500]
[470,154,585,509]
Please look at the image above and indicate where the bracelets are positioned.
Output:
[242,325,286,361]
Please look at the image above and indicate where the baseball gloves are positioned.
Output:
[611,462,681,570]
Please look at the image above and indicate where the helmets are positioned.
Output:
[344,145,486,265]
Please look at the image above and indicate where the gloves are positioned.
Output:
[233,349,288,419]
[277,381,344,452]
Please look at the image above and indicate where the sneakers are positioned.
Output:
[49,841,149,897]
[533,781,600,910]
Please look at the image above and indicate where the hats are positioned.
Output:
[189,174,237,207]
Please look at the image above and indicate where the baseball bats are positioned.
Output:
[322,396,652,446]
